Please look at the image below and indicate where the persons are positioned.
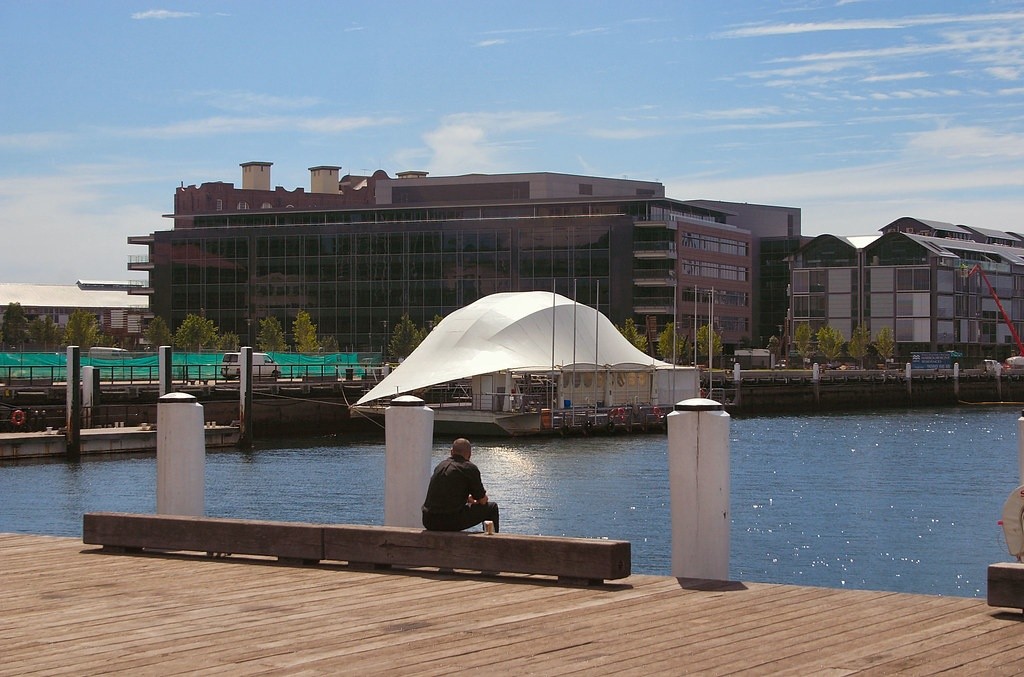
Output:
[421,438,500,533]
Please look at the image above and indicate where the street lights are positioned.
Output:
[56,353,61,376]
[778,325,783,360]
[10,343,22,376]
[380,321,389,365]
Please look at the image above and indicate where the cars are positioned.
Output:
[774,360,786,368]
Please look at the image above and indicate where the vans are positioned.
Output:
[220,353,281,380]
[89,347,132,360]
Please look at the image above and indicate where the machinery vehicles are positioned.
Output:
[969,264,1024,370]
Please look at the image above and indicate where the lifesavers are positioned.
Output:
[584,420,594,432]
[12,409,25,426]
[1001,483,1024,557]
[607,421,616,433]
[559,424,570,437]
[653,405,661,418]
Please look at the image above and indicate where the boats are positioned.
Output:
[958,399,1024,410]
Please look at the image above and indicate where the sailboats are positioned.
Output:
[347,278,739,438]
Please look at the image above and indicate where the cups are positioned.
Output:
[483,520,494,535]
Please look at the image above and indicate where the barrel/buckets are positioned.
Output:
[564,399,571,409]
[597,400,603,408]
[541,408,552,429]
